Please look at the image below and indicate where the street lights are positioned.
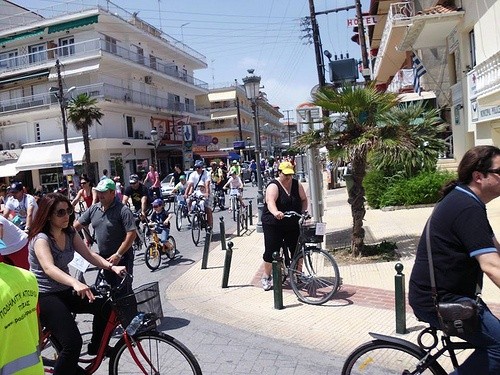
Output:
[59,85,77,201]
[247,137,252,161]
[149,127,160,174]
[242,68,266,233]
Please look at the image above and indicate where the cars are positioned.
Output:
[158,171,192,201]
[240,163,251,180]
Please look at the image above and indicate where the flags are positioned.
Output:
[411,52,426,96]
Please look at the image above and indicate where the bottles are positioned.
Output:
[126,311,147,335]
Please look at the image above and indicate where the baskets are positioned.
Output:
[302,222,326,244]
[114,281,164,332]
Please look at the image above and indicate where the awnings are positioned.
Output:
[16,140,86,171]
[193,152,230,171]
[0,160,21,177]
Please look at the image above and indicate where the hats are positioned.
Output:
[193,160,204,167]
[228,170,236,176]
[92,178,116,192]
[128,173,138,183]
[197,181,205,188]
[278,162,294,175]
[179,175,185,180]
[151,199,164,205]
[231,160,237,164]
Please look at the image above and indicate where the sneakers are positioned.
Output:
[260,275,272,292]
[297,273,310,286]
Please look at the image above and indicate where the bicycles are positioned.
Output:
[341,316,476,375]
[71,201,95,247]
[171,182,243,246]
[141,222,177,271]
[264,210,340,306]
[39,263,202,375]
[125,187,171,261]
[247,169,275,188]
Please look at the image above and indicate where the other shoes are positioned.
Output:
[227,208,232,213]
[200,210,205,214]
[189,211,196,215]
[241,202,245,208]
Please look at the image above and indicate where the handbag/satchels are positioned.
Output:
[434,301,477,336]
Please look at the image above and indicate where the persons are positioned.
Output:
[124,164,174,258]
[209,160,246,210]
[0,169,137,375]
[261,162,309,292]
[172,160,214,232]
[408,146,500,375]
[248,156,296,182]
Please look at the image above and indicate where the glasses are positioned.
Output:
[130,182,137,184]
[479,167,500,177]
[54,208,73,216]
[80,182,87,185]
[153,205,161,208]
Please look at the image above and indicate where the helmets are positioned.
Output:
[210,162,218,166]
[8,183,23,192]
[219,161,225,168]
